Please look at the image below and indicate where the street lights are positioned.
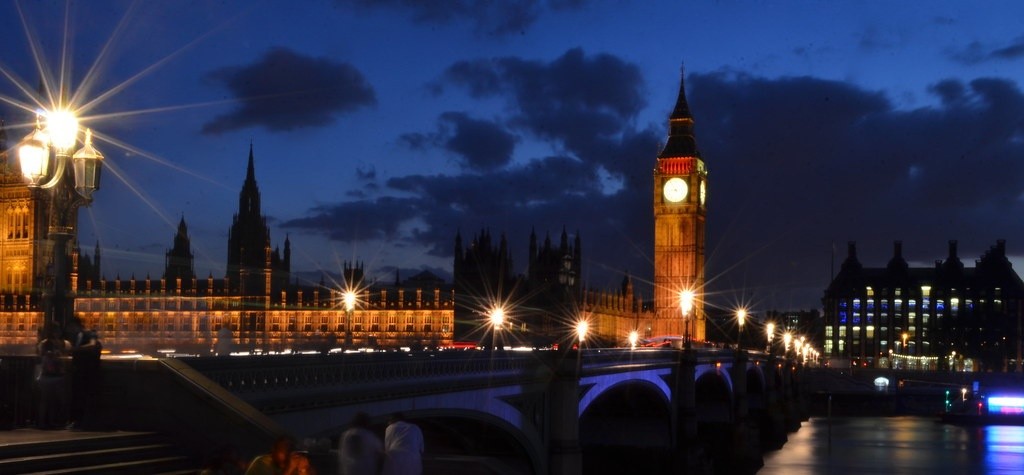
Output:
[733,306,749,351]
[575,319,592,352]
[483,305,506,354]
[20,85,104,343]
[677,287,696,353]
[765,322,777,360]
[340,287,358,352]
